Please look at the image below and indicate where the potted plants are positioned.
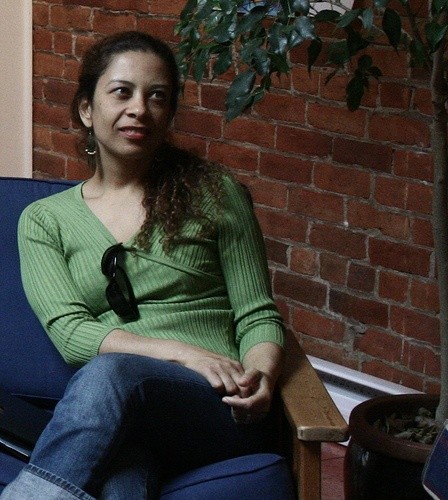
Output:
[170,0,448,500]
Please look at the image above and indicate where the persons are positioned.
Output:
[1,30,287,499]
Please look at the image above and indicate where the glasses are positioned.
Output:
[100,242,141,324]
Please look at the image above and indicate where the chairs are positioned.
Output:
[0,175,348,499]
